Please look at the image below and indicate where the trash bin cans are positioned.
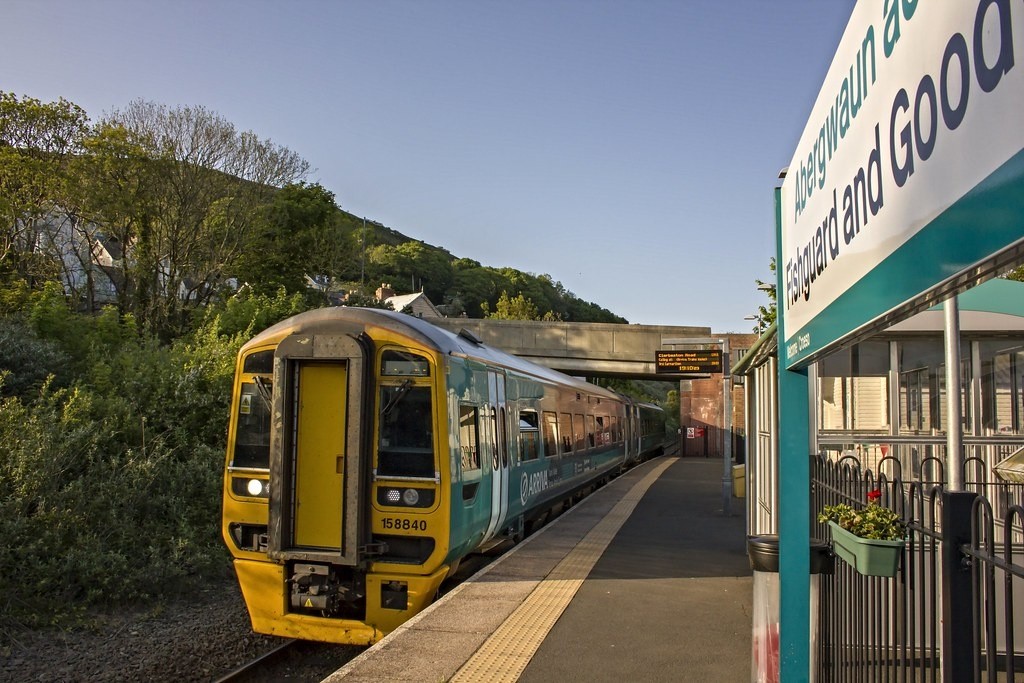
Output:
[746,534,836,683]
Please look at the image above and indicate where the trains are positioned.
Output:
[220,306,666,647]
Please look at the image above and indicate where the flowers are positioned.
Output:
[817,491,906,542]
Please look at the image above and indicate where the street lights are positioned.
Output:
[744,313,762,339]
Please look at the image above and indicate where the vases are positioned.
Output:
[825,520,904,577]
